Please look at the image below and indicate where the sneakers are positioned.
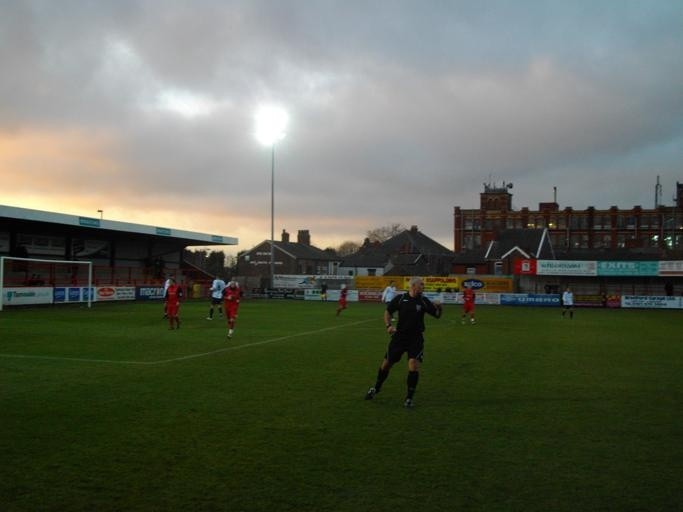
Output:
[364,385,380,399]
[403,395,413,408]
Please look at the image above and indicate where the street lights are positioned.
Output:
[258,107,286,295]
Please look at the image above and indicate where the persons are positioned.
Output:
[162,274,177,318]
[364,277,444,409]
[336,282,349,316]
[319,279,329,302]
[163,280,183,330]
[226,276,239,288]
[381,280,397,322]
[221,282,241,338]
[204,272,225,320]
[461,281,477,325]
[560,287,576,320]
